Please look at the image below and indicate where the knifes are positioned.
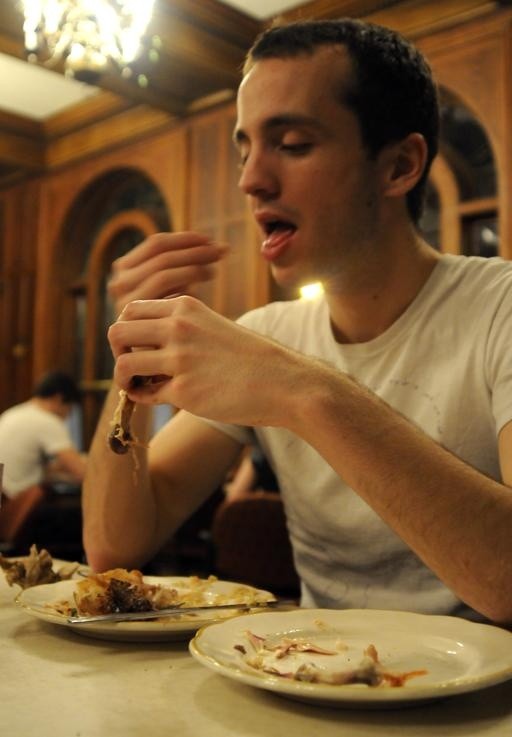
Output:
[62,602,274,625]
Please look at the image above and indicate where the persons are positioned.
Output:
[0,367,88,500]
[222,442,282,510]
[73,13,511,633]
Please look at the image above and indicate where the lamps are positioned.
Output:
[22,1,154,70]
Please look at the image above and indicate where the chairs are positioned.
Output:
[0,464,88,567]
[212,493,302,606]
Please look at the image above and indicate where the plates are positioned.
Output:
[15,575,280,646]
[189,607,512,707]
